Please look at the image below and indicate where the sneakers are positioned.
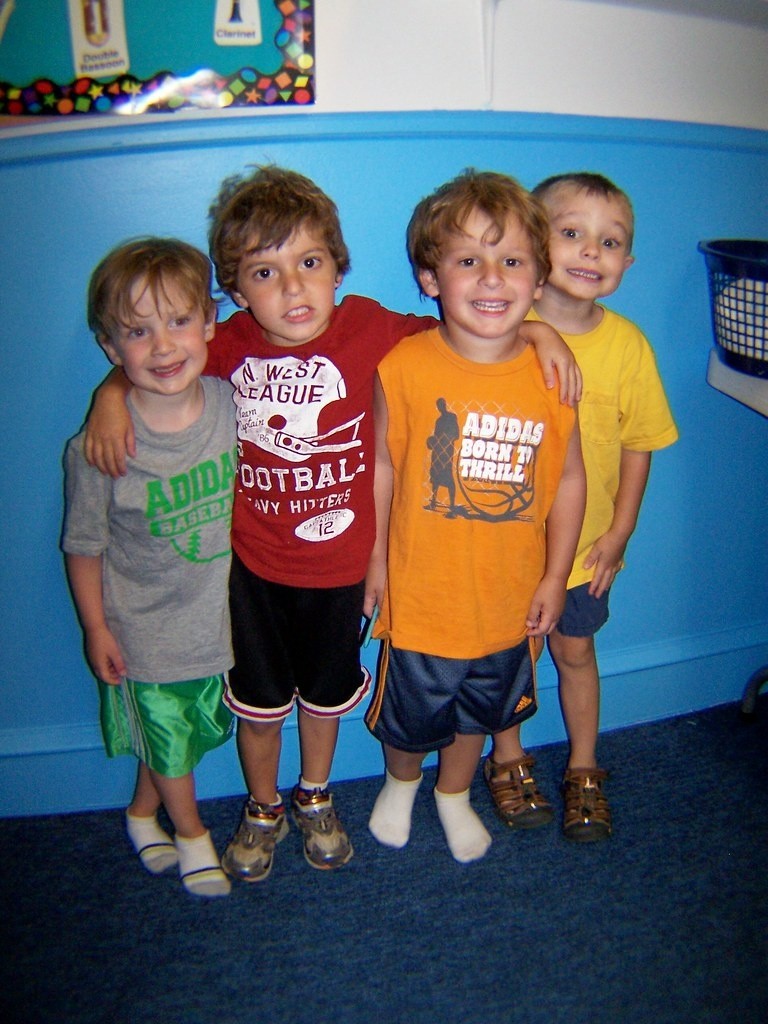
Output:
[221,786,290,882]
[289,783,355,870]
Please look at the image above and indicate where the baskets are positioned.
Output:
[697,238,768,379]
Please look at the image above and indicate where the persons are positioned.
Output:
[84,163,583,882]
[362,172,587,862]
[484,172,679,843]
[61,237,240,898]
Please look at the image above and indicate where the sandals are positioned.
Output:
[485,751,555,829]
[563,768,613,844]
